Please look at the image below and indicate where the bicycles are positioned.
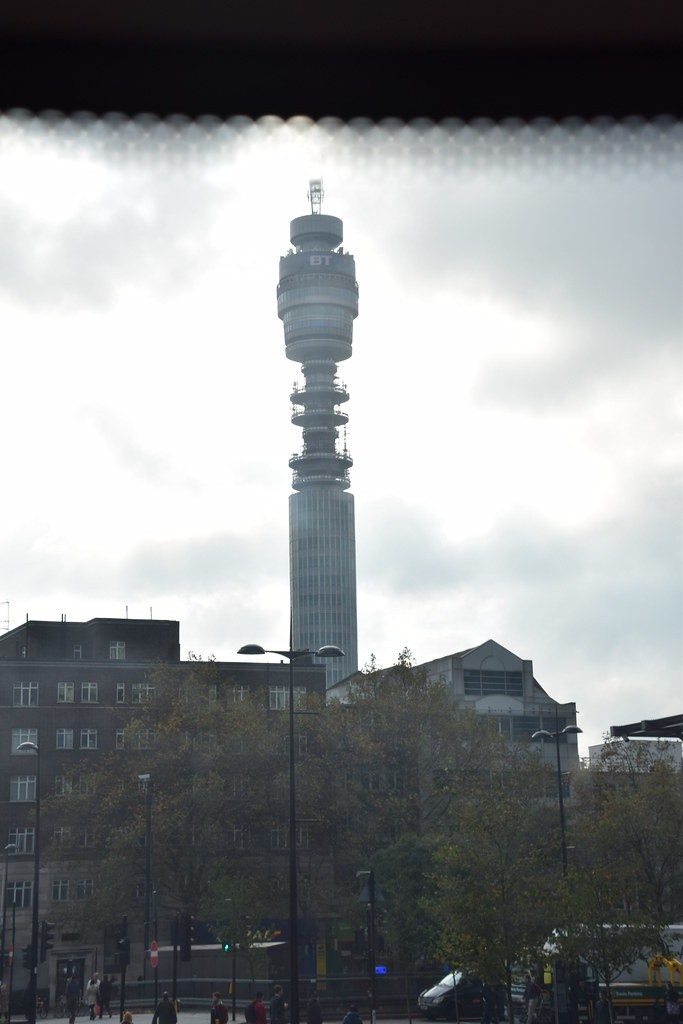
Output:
[39,990,87,1019]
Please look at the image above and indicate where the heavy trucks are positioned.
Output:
[505,924,683,1024]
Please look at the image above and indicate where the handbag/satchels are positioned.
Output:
[243,1000,257,1024]
[94,1005,100,1016]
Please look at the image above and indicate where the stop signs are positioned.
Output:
[9,946,13,966]
[150,941,159,969]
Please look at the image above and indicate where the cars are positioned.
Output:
[417,971,483,1022]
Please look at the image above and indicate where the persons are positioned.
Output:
[63,974,82,1024]
[120,1011,134,1024]
[152,991,177,1024]
[85,972,100,1020]
[251,992,267,1024]
[480,982,500,1024]
[595,991,615,1024]
[522,975,541,1024]
[270,985,288,1024]
[307,991,323,1024]
[99,975,112,1018]
[341,1005,363,1024]
[211,992,228,1024]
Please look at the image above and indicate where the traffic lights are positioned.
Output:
[21,944,32,969]
[118,938,130,966]
[42,920,56,963]
[179,913,195,961]
[222,943,231,952]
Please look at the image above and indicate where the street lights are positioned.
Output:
[355,870,376,1024]
[17,742,41,1024]
[237,606,346,1024]
[0,844,19,981]
[530,702,583,1024]
